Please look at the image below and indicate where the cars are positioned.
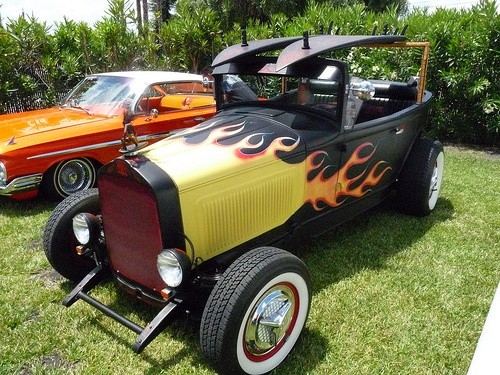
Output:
[41,21,446,375]
[0,71,268,204]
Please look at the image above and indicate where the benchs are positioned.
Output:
[157,93,214,113]
[354,80,418,125]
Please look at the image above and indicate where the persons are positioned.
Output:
[203,66,258,104]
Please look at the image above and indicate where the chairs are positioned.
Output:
[306,79,340,105]
